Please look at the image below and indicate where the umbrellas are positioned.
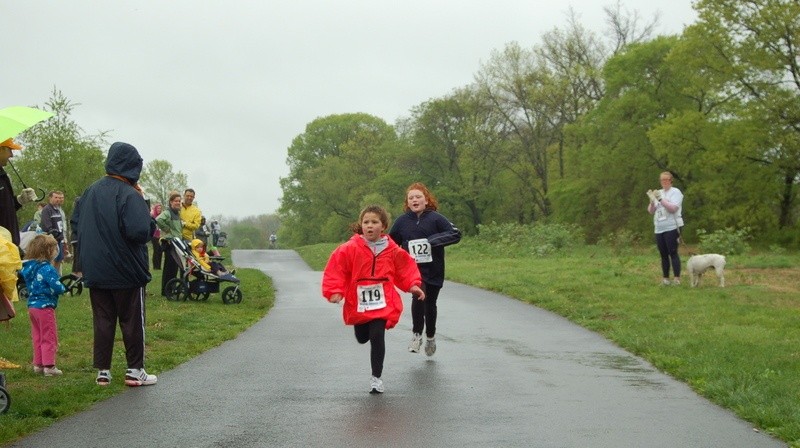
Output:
[0,105,56,201]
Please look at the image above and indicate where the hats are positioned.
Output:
[0,138,23,150]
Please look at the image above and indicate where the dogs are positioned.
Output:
[685,253,727,288]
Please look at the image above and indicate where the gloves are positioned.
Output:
[18,188,37,204]
[646,189,662,202]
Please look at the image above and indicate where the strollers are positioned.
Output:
[165,234,243,303]
[17,221,40,303]
[57,274,89,298]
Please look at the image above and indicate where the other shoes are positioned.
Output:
[368,376,384,393]
[409,332,423,353]
[222,269,235,275]
[0,356,21,369]
[425,337,436,356]
[658,279,681,288]
[34,365,63,377]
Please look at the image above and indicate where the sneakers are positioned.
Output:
[124,367,157,386]
[95,369,112,385]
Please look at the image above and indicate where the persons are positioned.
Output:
[322,206,425,394]
[388,183,462,355]
[647,171,684,287]
[0,138,279,387]
